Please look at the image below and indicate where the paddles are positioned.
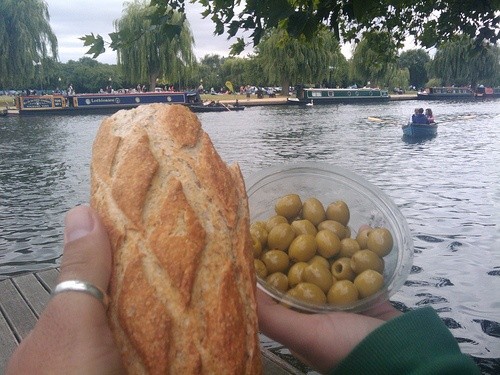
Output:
[430,114,476,124]
[366,116,408,126]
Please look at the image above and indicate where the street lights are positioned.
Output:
[200,78,203,93]
[108,77,111,92]
[58,76,61,91]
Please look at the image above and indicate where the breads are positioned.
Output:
[86,103,263,375]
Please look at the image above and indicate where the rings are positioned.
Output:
[50,280,110,313]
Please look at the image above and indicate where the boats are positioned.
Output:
[288,89,390,105]
[0,92,204,115]
[474,86,500,101]
[402,122,438,138]
[191,102,244,112]
[417,86,477,98]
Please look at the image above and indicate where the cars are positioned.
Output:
[0,90,61,96]
[118,88,162,93]
[394,87,404,94]
[240,86,281,95]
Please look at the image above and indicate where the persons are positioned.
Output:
[256,288,482,375]
[6,204,125,375]
[411,108,435,124]
[1,78,486,108]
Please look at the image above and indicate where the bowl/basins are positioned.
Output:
[238,158,414,313]
